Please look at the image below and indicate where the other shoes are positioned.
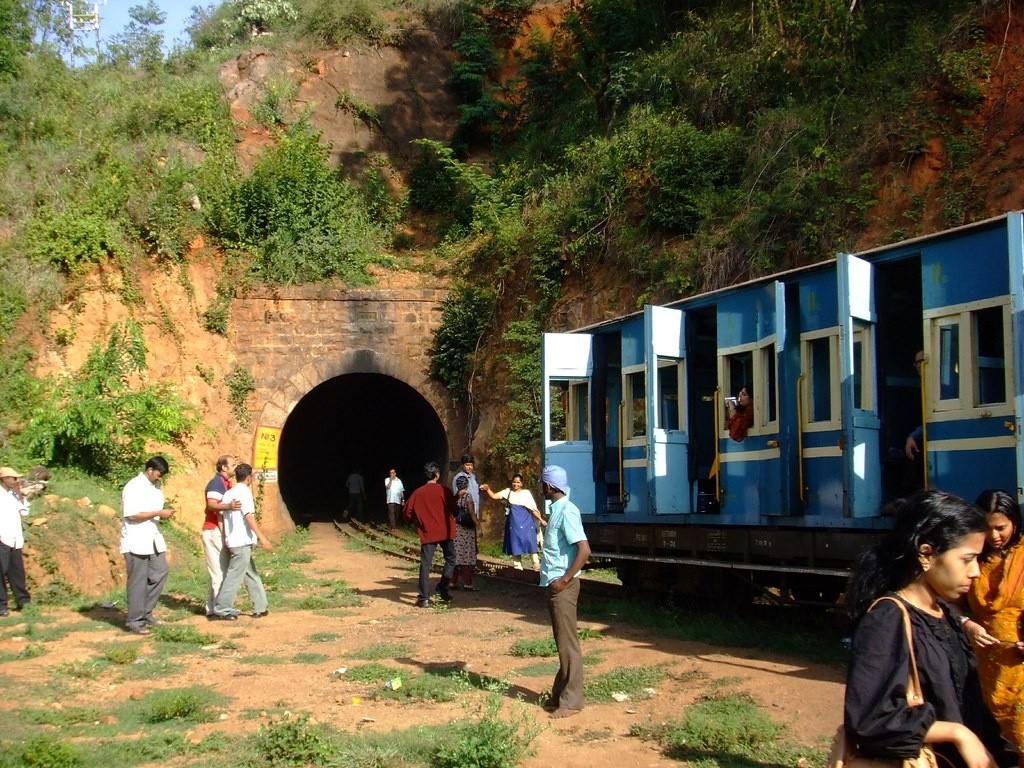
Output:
[0,611,8,616]
[254,610,268,617]
[343,510,347,518]
[214,613,237,620]
[418,599,430,608]
[533,564,541,572]
[437,591,452,601]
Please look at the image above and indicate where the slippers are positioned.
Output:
[450,584,460,590]
[463,585,479,591]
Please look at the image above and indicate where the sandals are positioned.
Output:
[146,618,162,626]
[128,625,149,634]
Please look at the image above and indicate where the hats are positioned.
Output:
[0,467,23,478]
[542,465,568,493]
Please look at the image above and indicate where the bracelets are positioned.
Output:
[730,406,736,412]
[959,615,970,628]
[402,499,405,502]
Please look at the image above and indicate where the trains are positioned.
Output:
[539,208,1024,634]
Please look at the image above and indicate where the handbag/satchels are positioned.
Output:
[828,597,938,768]
[504,489,511,516]
[458,493,474,528]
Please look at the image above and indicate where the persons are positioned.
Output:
[938,490,1024,768]
[882,347,925,516]
[202,455,243,621]
[385,468,405,532]
[213,464,273,620]
[452,454,479,573]
[120,456,174,635]
[448,475,482,591]
[538,464,592,718]
[342,470,367,522]
[403,462,460,607]
[725,385,754,443]
[480,473,548,573]
[844,487,1006,768]
[0,467,32,617]
